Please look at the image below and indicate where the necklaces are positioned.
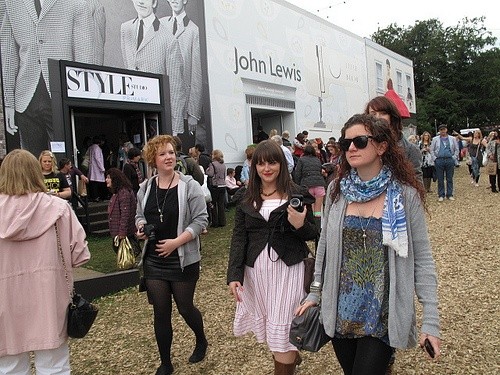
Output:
[356,195,380,249]
[262,190,277,196]
[155,173,175,222]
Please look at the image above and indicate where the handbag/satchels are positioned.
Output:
[288,305,332,353]
[81,155,89,171]
[484,158,497,176]
[207,162,218,187]
[67,292,99,340]
[113,233,139,272]
[200,174,213,204]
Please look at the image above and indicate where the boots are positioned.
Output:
[272,350,303,375]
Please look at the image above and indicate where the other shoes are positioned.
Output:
[188,336,208,363]
[448,197,455,201]
[437,197,444,203]
[471,179,480,187]
[154,362,175,375]
[209,224,225,228]
[201,229,208,234]
[492,187,496,192]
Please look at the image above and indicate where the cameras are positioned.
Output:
[289,193,305,212]
[140,224,155,240]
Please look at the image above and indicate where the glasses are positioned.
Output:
[339,135,379,153]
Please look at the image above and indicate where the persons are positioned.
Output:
[0,0,107,159]
[172,136,257,227]
[257,126,344,233]
[59,158,89,211]
[121,0,186,136]
[407,125,500,201]
[366,96,424,187]
[86,137,148,201]
[294,114,441,375]
[226,140,319,375]
[39,150,71,198]
[0,150,90,374]
[158,0,201,151]
[135,135,208,375]
[106,169,137,252]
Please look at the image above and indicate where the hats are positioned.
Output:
[438,124,447,129]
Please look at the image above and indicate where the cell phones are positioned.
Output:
[425,338,434,358]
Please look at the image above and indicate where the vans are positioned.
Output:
[460,127,483,148]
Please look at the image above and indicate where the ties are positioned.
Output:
[34,0,41,16]
[172,18,178,36]
[137,20,144,48]
